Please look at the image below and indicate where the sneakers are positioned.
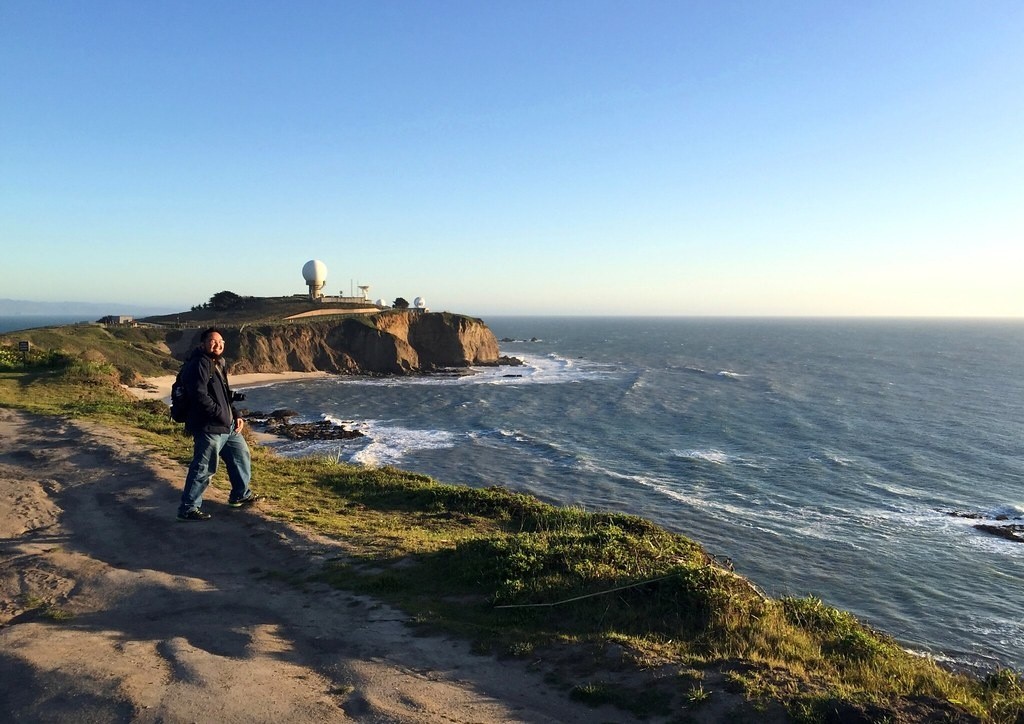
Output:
[176,509,212,522]
[228,494,265,507]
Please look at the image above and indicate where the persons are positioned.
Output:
[176,330,259,521]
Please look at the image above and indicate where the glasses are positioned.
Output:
[205,339,226,344]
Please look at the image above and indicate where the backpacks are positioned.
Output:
[170,353,216,423]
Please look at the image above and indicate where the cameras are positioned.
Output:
[228,391,246,403]
